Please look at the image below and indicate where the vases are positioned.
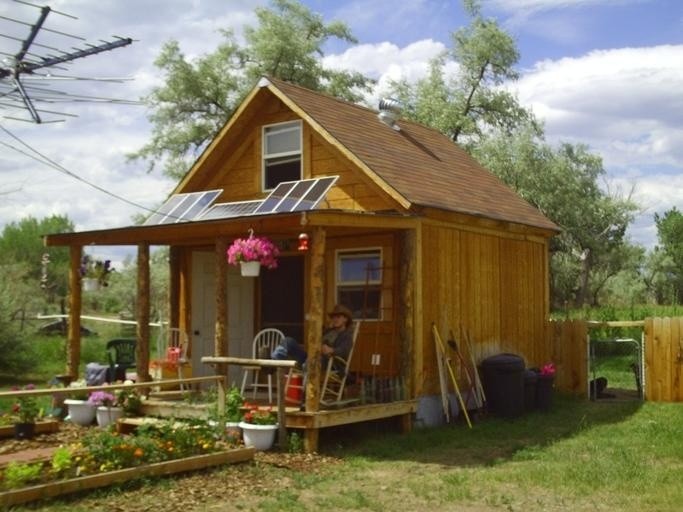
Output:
[63,399,97,426]
[239,260,261,277]
[80,277,98,292]
[239,420,279,451]
[96,406,122,428]
[13,422,35,440]
[116,420,136,435]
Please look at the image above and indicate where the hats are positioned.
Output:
[327,304,352,319]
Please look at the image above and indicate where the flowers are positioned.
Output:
[10,384,39,424]
[77,255,115,287]
[243,400,277,425]
[70,379,89,400]
[227,236,280,270]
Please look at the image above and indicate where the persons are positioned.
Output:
[262,304,353,374]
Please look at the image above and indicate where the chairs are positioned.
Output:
[236,330,286,404]
[157,328,189,391]
[105,339,137,382]
[284,318,361,405]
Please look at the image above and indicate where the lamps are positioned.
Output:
[297,232,309,254]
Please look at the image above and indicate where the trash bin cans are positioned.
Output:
[540,376,553,410]
[479,353,526,418]
[524,368,539,411]
